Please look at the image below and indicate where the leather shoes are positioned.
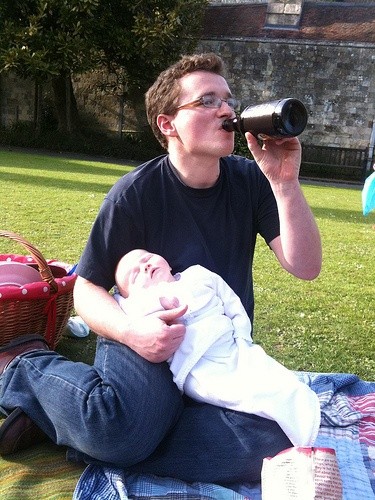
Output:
[0,334,50,376]
[0,407,54,455]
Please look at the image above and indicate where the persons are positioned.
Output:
[109,246,320,449]
[0,53,322,487]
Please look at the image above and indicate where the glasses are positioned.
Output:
[166,95,240,115]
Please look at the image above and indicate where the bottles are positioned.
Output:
[223,98,308,138]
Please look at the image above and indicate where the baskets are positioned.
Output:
[0,230,78,351]
